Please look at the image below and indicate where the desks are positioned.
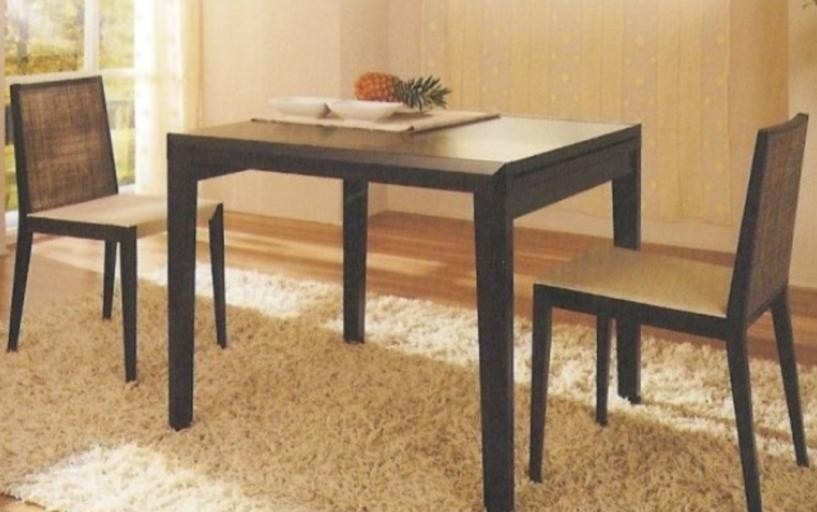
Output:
[155,107,642,512]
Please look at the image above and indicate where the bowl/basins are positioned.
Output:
[327,99,404,121]
[267,96,329,117]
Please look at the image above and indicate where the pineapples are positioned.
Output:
[355,73,451,112]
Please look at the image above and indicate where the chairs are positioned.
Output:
[11,71,226,383]
[528,109,810,512]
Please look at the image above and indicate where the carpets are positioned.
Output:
[0,259,812,512]
